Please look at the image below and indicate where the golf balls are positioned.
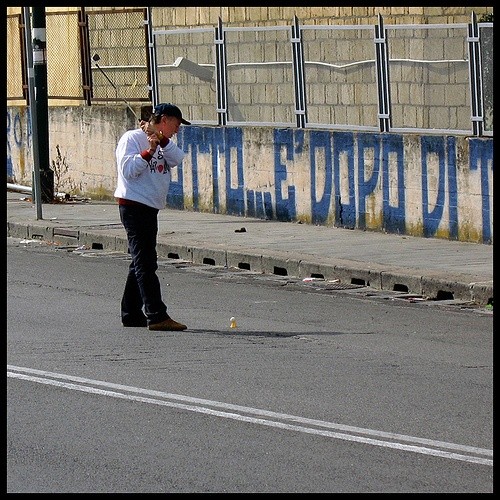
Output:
[230,317,236,322]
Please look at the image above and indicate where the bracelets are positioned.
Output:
[147,148,155,153]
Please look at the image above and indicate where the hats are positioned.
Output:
[154,103,191,125]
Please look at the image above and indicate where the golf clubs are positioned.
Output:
[91,54,141,123]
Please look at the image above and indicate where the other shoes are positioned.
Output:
[149,318,187,331]
[121,310,151,326]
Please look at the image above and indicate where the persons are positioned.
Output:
[114,102,194,333]
